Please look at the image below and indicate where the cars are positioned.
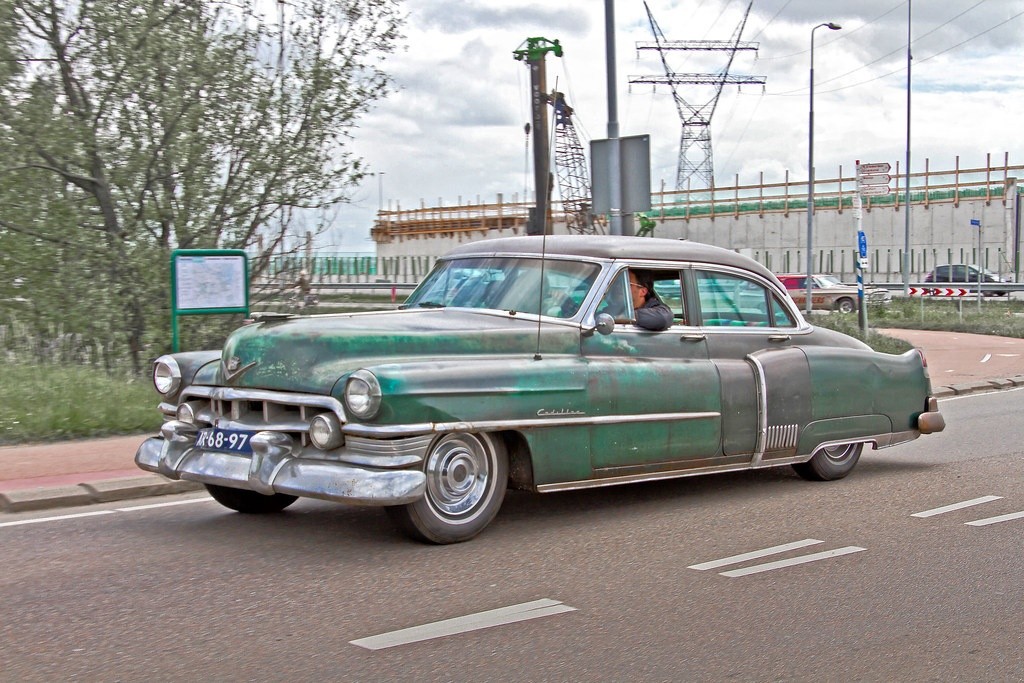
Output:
[740,273,893,315]
[924,263,1011,296]
[131,235,947,547]
[805,21,841,314]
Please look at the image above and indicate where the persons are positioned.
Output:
[293,268,312,294]
[550,89,568,107]
[118,288,148,385]
[554,267,677,331]
